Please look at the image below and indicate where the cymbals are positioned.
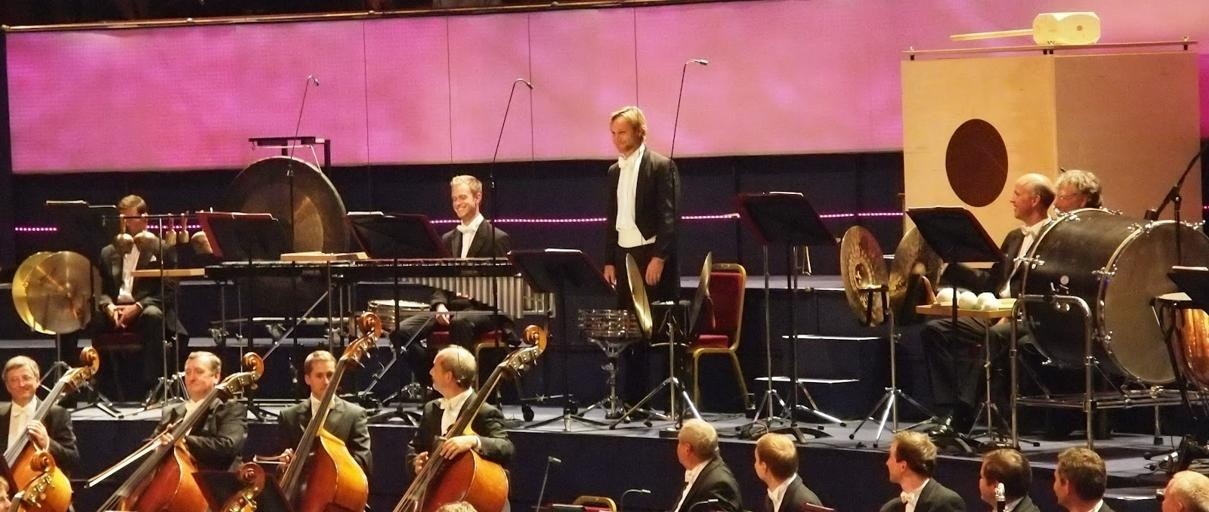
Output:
[840,225,888,326]
[27,252,102,331]
[626,252,654,341]
[689,250,711,337]
[10,251,60,326]
[889,225,944,318]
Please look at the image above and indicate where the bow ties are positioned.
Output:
[618,157,638,170]
[311,401,336,413]
[439,397,460,410]
[767,489,779,501]
[901,492,916,503]
[684,472,695,482]
[12,406,33,416]
[456,225,476,233]
[185,399,203,410]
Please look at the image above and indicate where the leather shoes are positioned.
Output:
[1021,226,1040,237]
[1163,443,1207,467]
[918,412,960,445]
[632,405,665,420]
[912,415,943,433]
[418,398,437,410]
[605,405,632,419]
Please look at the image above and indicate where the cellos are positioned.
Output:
[276,312,383,512]
[393,324,547,512]
[98,352,265,512]
[0,348,101,512]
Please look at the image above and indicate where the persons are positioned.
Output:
[405,343,517,489]
[978,448,1040,512]
[1161,470,1209,512]
[391,174,515,405]
[602,106,682,421]
[673,418,742,512]
[1053,447,1116,512]
[61,194,176,408]
[0,352,80,512]
[877,430,967,512]
[278,349,373,500]
[749,432,825,512]
[136,351,249,504]
[918,171,1056,450]
[984,167,1109,441]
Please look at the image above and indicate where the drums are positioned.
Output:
[1020,208,1209,386]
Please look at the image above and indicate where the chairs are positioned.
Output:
[425,317,535,425]
[571,493,619,512]
[679,260,753,416]
